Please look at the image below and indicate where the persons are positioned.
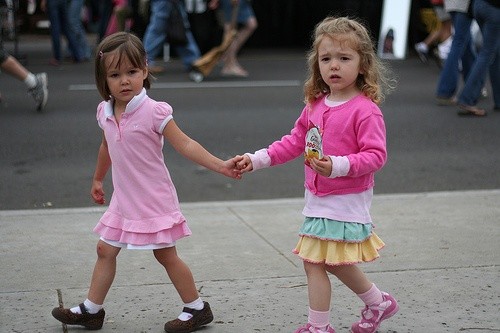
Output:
[417,0,500,116]
[44,0,258,82]
[0,45,49,112]
[234,17,401,333]
[51,32,246,333]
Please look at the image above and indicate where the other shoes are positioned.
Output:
[415,42,427,63]
[457,105,486,117]
[351,291,400,333]
[27,73,48,110]
[223,66,248,77]
[294,323,335,333]
[164,301,214,333]
[434,97,458,106]
[52,303,106,329]
